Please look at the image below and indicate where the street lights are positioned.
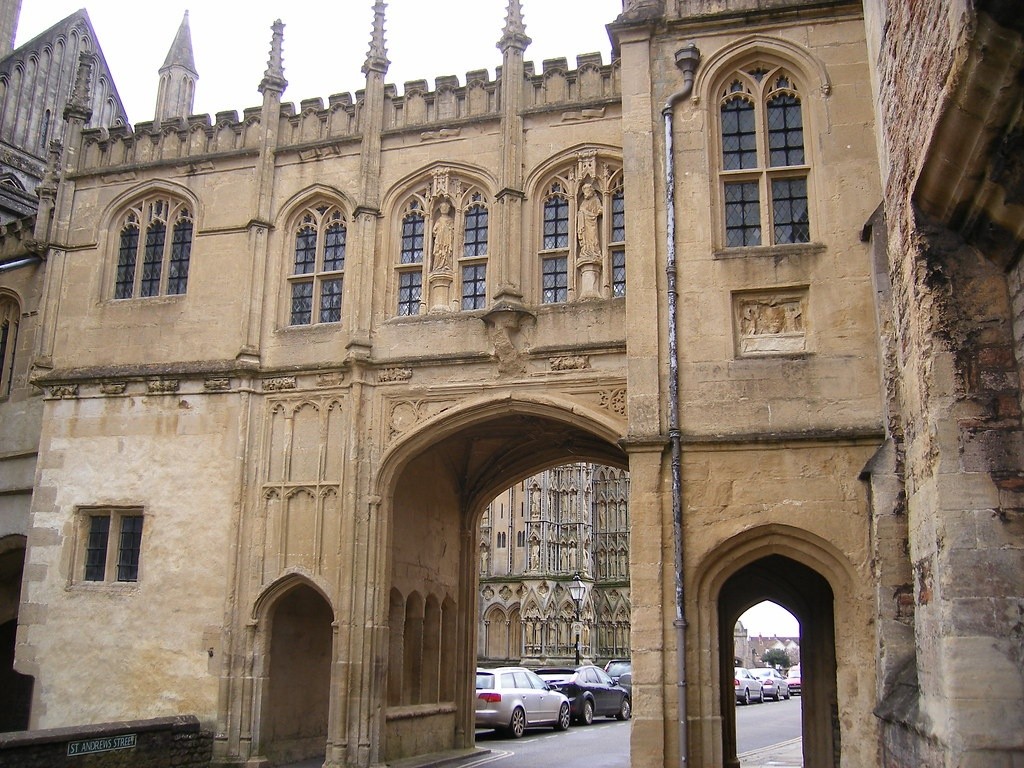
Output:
[566,572,587,664]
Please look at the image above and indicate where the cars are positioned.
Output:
[472,666,572,740]
[733,666,765,705]
[531,663,633,726]
[602,658,631,683]
[786,667,802,695]
[747,668,791,701]
